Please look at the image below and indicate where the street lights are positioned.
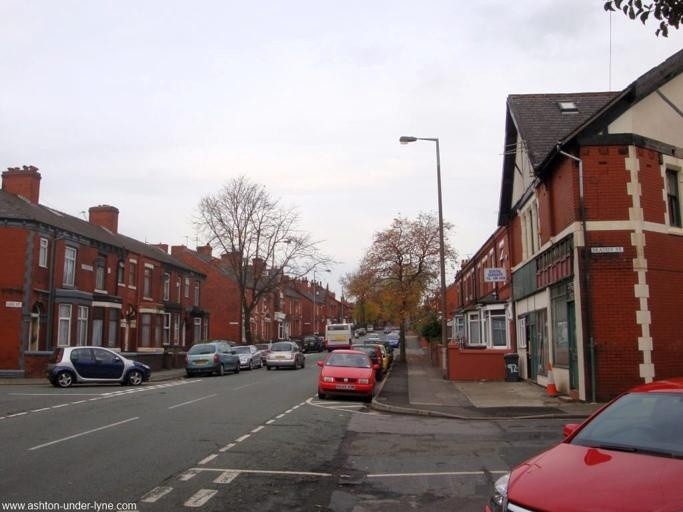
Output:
[312,269,331,332]
[398,135,451,380]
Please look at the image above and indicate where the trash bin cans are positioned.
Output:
[504,354,518,382]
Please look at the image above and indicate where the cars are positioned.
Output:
[46,345,152,388]
[298,322,375,354]
[316,327,402,403]
[482,375,682,512]
[183,339,305,375]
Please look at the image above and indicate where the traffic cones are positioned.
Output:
[543,361,559,397]
